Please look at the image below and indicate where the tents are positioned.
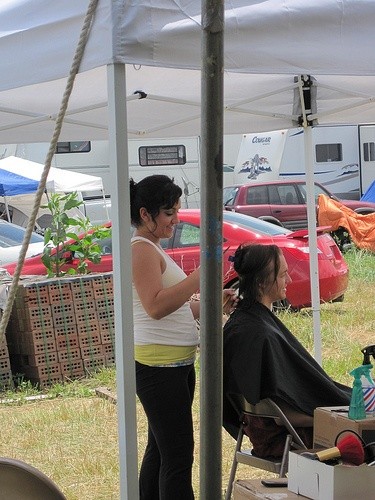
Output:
[0,1,375,500]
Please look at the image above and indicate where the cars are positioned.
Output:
[0,218,62,264]
[0,208,348,315]
[223,180,375,232]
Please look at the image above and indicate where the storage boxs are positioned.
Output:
[288,449,375,500]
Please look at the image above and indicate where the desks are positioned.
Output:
[313,405,375,451]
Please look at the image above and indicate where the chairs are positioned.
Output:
[286,193,293,204]
[0,458,68,500]
[225,392,308,500]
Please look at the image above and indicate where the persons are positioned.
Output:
[129,175,240,500]
[223,244,353,462]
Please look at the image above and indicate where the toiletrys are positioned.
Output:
[361,345,375,411]
[348,364,374,419]
